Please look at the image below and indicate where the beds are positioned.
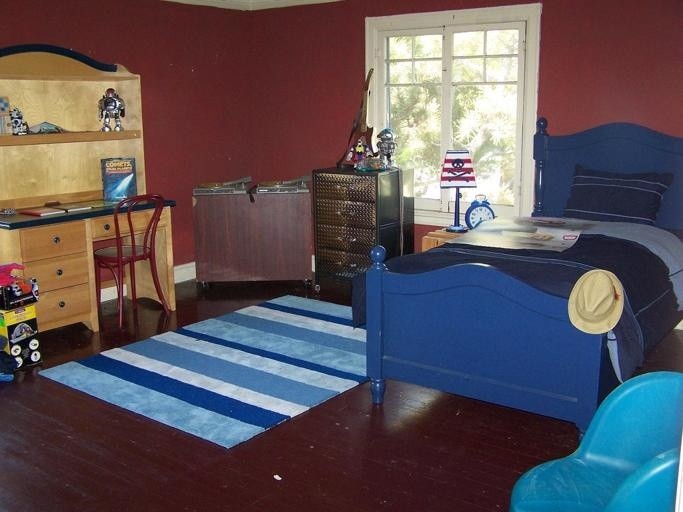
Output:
[366,117,683,445]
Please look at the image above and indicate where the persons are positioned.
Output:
[529,218,567,225]
[488,226,554,242]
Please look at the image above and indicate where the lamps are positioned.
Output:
[440,150,477,233]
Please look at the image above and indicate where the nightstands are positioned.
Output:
[422,228,464,251]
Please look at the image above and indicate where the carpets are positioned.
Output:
[38,294,370,450]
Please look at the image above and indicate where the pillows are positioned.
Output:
[564,164,672,225]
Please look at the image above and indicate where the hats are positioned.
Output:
[566,268,625,336]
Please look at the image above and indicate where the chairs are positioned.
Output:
[93,194,170,327]
[509,370,683,512]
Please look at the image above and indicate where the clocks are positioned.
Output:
[465,195,494,230]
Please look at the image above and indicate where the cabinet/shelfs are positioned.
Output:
[0,44,176,334]
[193,194,315,293]
[312,167,400,296]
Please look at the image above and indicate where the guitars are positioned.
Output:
[340,69,374,169]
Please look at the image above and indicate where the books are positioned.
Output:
[100,157,137,202]
[52,202,92,212]
[16,206,65,218]
